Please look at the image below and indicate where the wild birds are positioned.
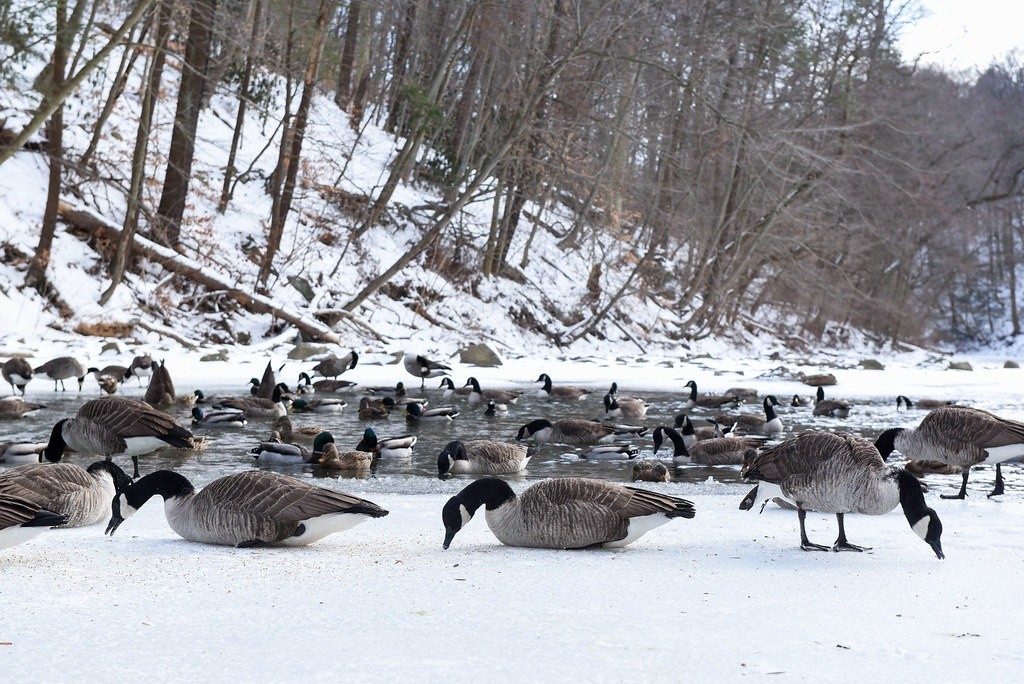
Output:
[0,340,1024,561]
